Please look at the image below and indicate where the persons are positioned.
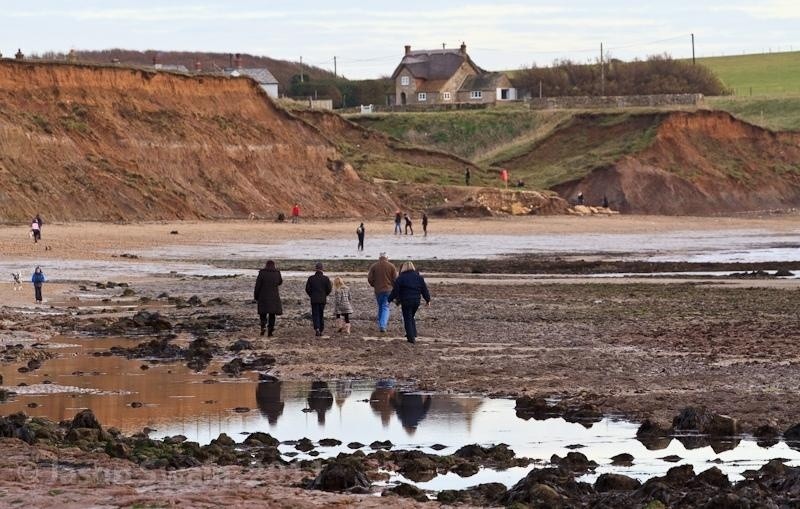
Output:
[466,167,470,186]
[32,266,45,304]
[254,376,433,435]
[394,208,428,237]
[31,219,40,242]
[305,263,333,335]
[333,277,353,335]
[500,167,508,189]
[356,223,364,250]
[517,179,524,187]
[368,251,430,344]
[291,203,300,224]
[602,194,609,208]
[254,260,282,336]
[35,215,42,239]
[576,190,583,205]
